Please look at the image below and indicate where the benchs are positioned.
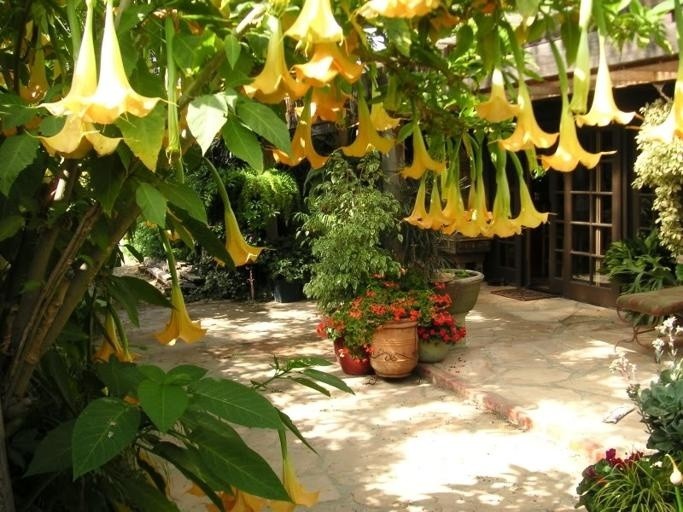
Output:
[614,285,682,358]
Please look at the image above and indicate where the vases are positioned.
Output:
[415,339,454,364]
[365,320,422,381]
[436,269,485,313]
[328,333,371,377]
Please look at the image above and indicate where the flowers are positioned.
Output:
[314,271,466,350]
